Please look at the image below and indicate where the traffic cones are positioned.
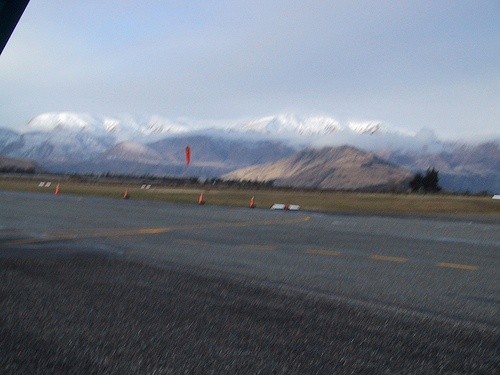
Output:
[199,191,205,205]
[54,184,60,195]
[123,190,130,199]
[249,195,255,210]
